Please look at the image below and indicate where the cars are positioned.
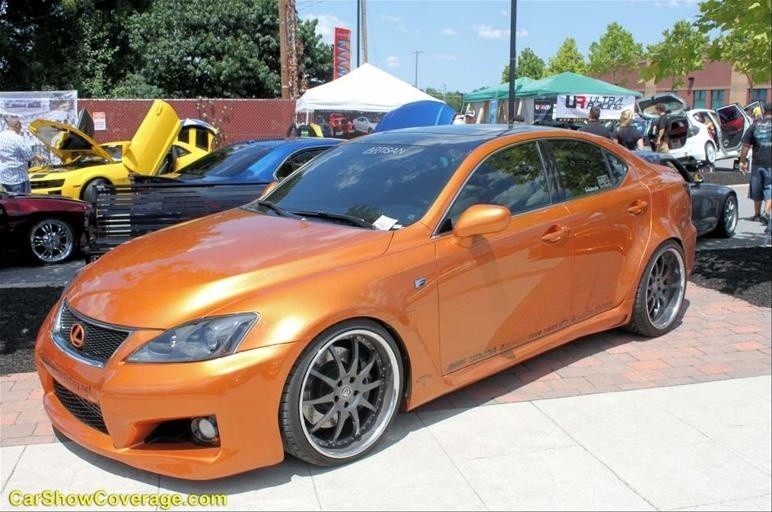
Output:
[352,116,381,135]
[744,99,767,123]
[121,97,457,239]
[31,121,699,484]
[0,187,99,265]
[633,93,754,169]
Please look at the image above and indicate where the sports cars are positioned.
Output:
[25,108,227,221]
[626,146,740,240]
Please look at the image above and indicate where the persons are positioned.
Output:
[577,107,610,139]
[653,103,671,152]
[739,103,771,226]
[611,109,643,152]
[0,118,46,195]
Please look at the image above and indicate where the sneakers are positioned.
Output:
[751,211,770,225]
